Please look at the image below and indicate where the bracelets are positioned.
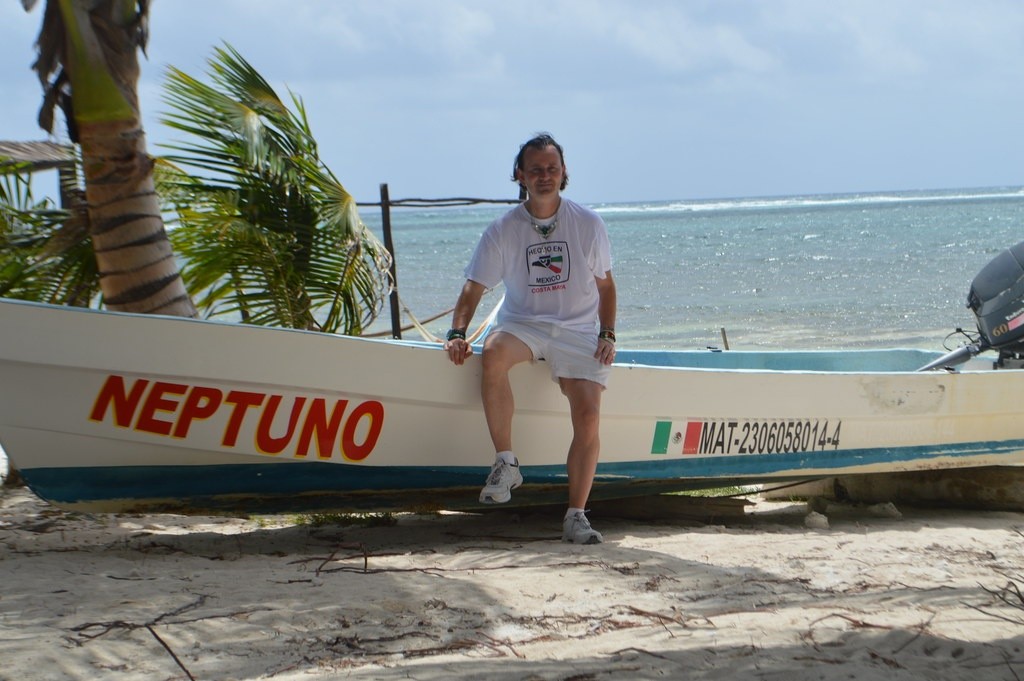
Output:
[445,329,465,341]
[597,327,616,347]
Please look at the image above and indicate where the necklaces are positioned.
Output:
[527,197,561,239]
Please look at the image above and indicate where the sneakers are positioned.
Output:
[562,509,603,544]
[478,456,523,503]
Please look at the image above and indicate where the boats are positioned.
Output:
[0,238,1023,513]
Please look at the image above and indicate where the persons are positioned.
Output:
[443,130,618,545]
